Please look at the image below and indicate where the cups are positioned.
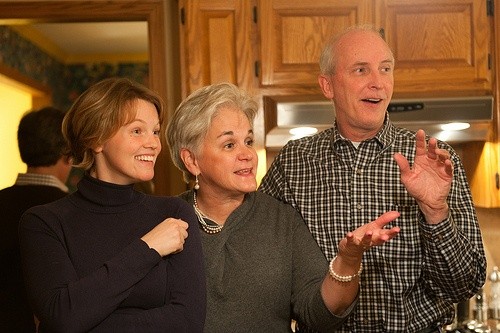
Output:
[443,303,457,331]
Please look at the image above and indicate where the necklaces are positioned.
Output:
[193,191,223,234]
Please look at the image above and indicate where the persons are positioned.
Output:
[165,81,401,333]
[0,106,78,333]
[257,26,487,333]
[18,77,206,333]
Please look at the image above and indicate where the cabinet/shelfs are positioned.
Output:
[186,0,491,191]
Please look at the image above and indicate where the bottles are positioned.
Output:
[487,266,500,333]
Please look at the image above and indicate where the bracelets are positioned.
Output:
[328,253,363,283]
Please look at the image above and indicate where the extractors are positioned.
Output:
[266,96,494,150]
